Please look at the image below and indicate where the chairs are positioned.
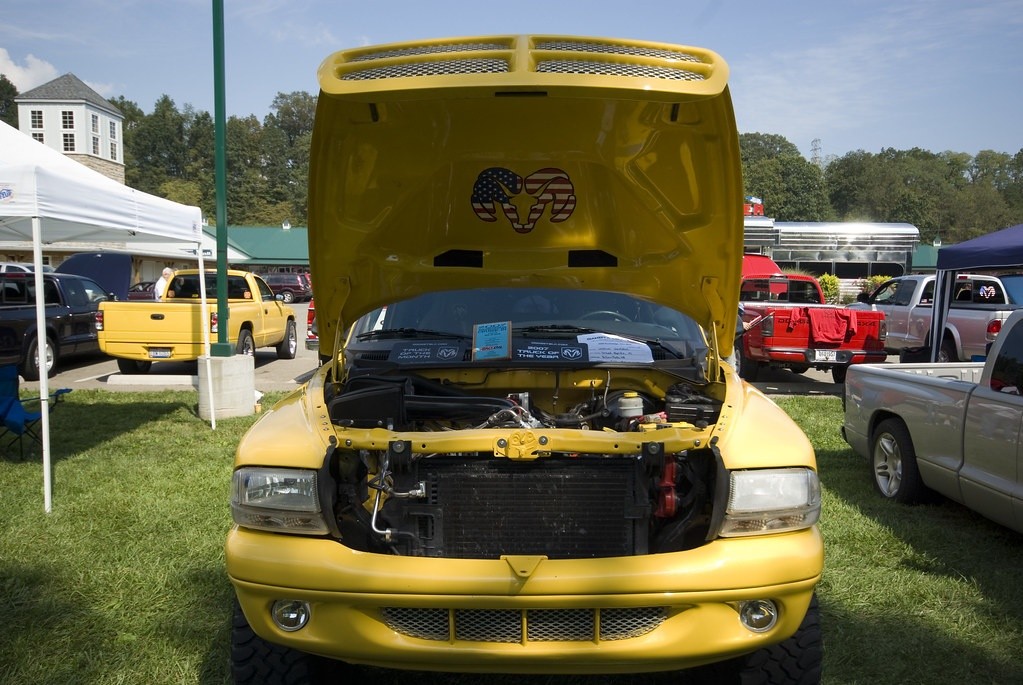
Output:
[0,287,20,298]
[957,289,972,301]
[0,364,73,460]
[777,292,788,301]
[181,283,198,297]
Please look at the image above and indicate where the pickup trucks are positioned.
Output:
[844,275,1023,363]
[733,251,888,385]
[0,251,132,380]
[842,307,1023,535]
[306,295,320,350]
[95,267,297,375]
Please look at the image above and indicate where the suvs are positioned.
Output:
[257,272,312,304]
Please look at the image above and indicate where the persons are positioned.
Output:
[154,267,184,301]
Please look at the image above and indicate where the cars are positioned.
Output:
[125,281,156,301]
[224,34,824,674]
[0,262,56,298]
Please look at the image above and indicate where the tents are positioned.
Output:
[931,224,1023,363]
[0,118,218,514]
